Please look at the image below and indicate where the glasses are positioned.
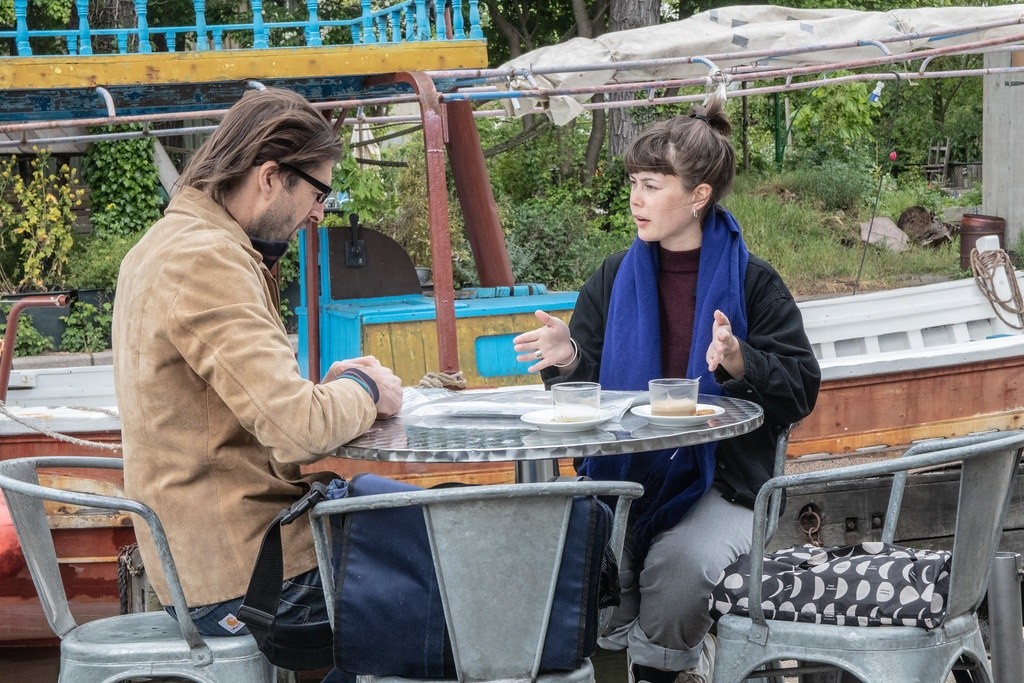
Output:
[251,159,332,204]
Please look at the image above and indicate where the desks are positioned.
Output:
[326,390,765,483]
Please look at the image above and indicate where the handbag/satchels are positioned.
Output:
[236,471,622,679]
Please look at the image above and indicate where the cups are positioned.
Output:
[551,380,602,424]
[648,377,699,417]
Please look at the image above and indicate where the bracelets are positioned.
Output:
[554,337,579,367]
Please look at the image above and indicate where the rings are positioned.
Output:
[535,349,543,358]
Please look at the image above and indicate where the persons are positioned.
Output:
[110,89,403,683]
[512,91,825,683]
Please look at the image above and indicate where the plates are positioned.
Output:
[520,407,615,433]
[630,404,726,427]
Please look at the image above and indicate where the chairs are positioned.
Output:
[917,135,952,187]
[604,420,801,683]
[0,454,297,683]
[708,428,1024,683]
[309,480,645,683]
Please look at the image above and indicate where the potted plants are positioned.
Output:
[0,143,155,348]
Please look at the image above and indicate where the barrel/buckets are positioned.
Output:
[959,214,1006,271]
